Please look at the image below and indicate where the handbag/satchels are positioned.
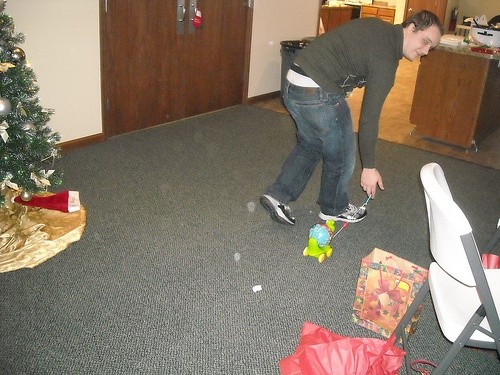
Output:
[351,248,429,349]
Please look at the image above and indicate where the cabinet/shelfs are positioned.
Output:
[361,6,396,25]
[409,50,500,149]
[320,9,351,32]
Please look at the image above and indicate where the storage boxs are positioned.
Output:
[456,25,470,37]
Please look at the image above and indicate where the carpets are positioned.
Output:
[0,105,500,375]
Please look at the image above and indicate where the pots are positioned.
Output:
[471,25,500,48]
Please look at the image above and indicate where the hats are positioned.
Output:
[11,191,80,213]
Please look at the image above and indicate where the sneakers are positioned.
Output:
[318,204,367,223]
[260,194,296,226]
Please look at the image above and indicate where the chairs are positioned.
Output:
[394,162,500,375]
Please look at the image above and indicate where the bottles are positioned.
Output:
[462,34,469,46]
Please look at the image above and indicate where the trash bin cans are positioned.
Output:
[280,39,311,99]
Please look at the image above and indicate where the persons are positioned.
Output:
[260,9,444,226]
